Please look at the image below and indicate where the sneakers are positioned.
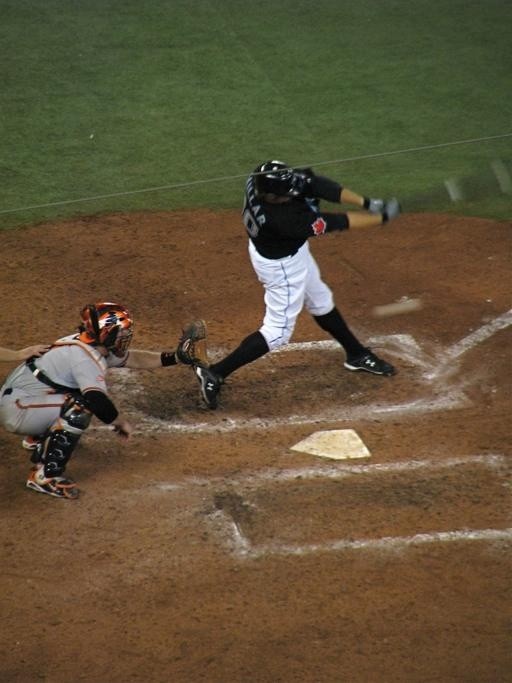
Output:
[197,366,224,409]
[27,467,79,499]
[344,348,394,376]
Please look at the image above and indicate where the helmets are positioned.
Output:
[255,160,312,197]
[78,301,134,358]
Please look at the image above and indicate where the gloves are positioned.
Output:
[364,197,400,224]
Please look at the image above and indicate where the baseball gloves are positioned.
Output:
[177,318,210,368]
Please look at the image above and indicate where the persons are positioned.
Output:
[196,161,395,410]
[1,301,211,499]
[1,341,50,362]
